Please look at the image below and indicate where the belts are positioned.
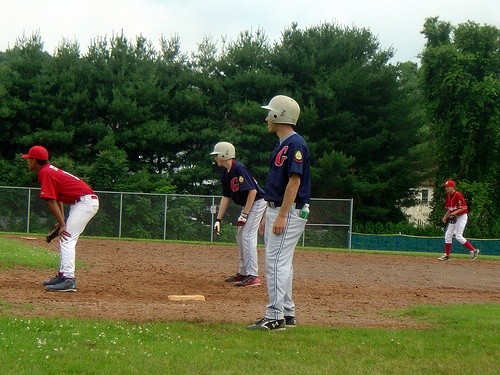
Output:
[269,201,304,210]
[73,196,97,204]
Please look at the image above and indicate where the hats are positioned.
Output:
[21,146,49,160]
[442,180,456,187]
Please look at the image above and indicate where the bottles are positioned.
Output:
[299,203,310,220]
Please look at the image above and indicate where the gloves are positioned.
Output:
[214,219,222,236]
[236,210,248,226]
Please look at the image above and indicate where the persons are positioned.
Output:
[437,180,480,262]
[209,141,268,288]
[21,145,100,291]
[247,95,312,331]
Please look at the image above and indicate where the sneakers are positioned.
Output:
[43,272,64,285]
[45,278,77,292]
[469,249,480,261]
[284,316,297,327]
[438,253,451,260]
[246,316,286,331]
[224,272,247,282]
[233,276,260,288]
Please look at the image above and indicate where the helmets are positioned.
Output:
[260,95,300,125]
[210,141,235,160]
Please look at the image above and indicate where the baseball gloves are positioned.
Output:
[46,223,61,243]
[446,213,457,224]
